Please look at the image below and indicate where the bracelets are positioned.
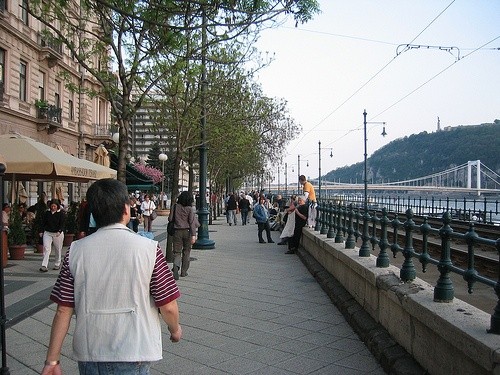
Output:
[45,360,60,366]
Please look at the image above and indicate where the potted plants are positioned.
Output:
[32,198,46,253]
[78,207,87,239]
[7,199,27,260]
[63,203,78,246]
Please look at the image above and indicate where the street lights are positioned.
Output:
[297,155,309,195]
[268,162,295,197]
[318,140,334,200]
[159,154,168,209]
[362,109,388,212]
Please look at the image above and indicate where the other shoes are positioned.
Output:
[277,241,288,244]
[181,273,188,277]
[284,248,296,254]
[173,266,180,280]
[53,266,60,270]
[268,240,275,243]
[39,267,48,272]
[259,241,266,243]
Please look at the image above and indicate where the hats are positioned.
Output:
[48,199,60,209]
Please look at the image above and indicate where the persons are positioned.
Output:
[42,179,182,375]
[253,195,308,254]
[127,191,168,233]
[206,192,215,205]
[299,175,317,204]
[168,191,200,280]
[225,191,258,226]
[3,192,97,272]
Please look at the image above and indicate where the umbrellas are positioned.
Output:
[94,144,109,167]
[0,131,117,205]
[49,143,65,204]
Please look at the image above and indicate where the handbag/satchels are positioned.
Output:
[150,210,158,220]
[249,207,251,211]
[166,221,175,236]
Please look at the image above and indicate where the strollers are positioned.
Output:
[269,207,282,231]
